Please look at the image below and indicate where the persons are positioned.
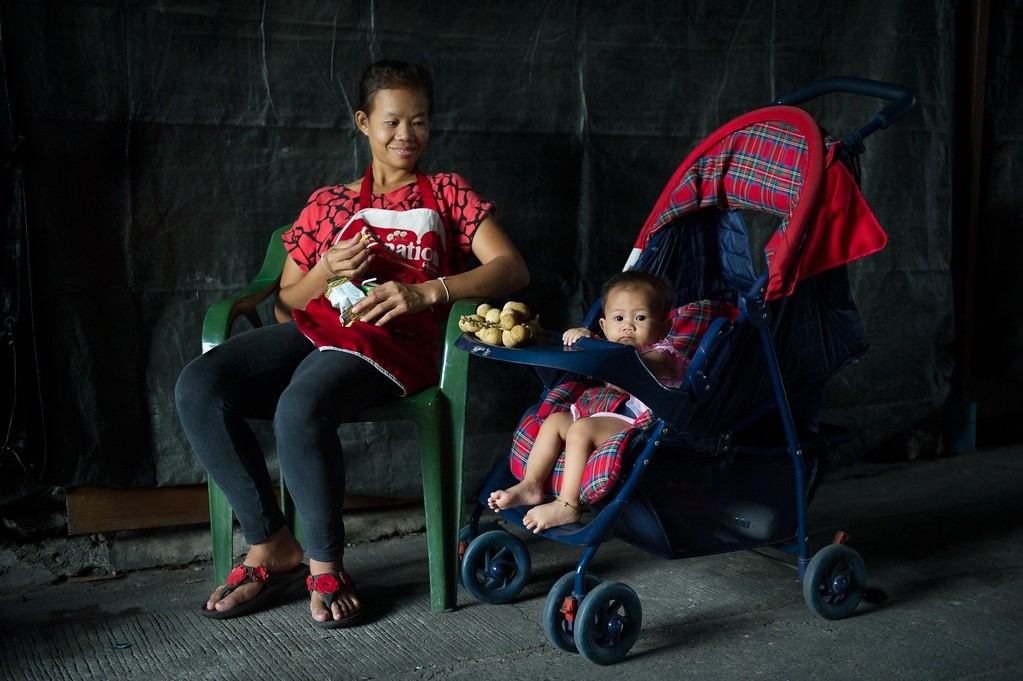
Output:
[487,269,695,534]
[174,60,528,630]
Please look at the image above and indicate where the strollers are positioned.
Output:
[452,101,890,668]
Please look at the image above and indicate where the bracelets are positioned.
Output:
[321,253,335,275]
[437,277,450,303]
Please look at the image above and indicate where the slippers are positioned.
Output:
[202,562,308,619]
[305,559,364,628]
